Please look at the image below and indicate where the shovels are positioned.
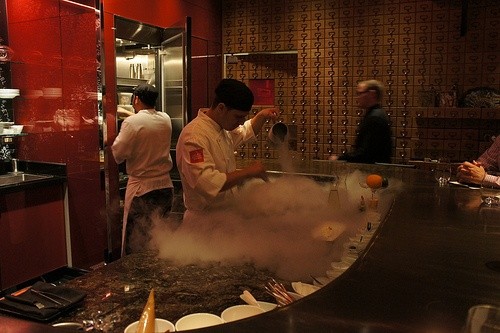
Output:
[8,295,61,310]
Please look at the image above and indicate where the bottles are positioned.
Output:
[129,64,141,79]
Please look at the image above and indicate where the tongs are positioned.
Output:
[31,288,73,307]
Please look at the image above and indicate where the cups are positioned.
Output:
[465,306,500,333]
[480,179,500,207]
[434,162,453,185]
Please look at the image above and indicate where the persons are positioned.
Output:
[110,83,176,259]
[457,135,500,188]
[326,80,393,164]
[175,78,282,224]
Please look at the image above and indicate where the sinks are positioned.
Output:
[0,174,54,187]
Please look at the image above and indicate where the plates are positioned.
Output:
[22,90,42,97]
[0,89,21,99]
[43,88,61,98]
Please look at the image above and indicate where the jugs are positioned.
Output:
[267,117,288,147]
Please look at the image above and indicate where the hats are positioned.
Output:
[215,79,254,111]
[134,83,159,101]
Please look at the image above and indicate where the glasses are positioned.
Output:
[355,89,369,95]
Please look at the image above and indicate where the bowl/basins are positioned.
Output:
[175,313,225,331]
[279,291,305,304]
[291,186,397,297]
[23,125,33,132]
[252,301,278,312]
[123,318,175,333]
[11,126,23,133]
[221,305,266,322]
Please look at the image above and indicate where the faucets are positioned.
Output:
[9,158,24,174]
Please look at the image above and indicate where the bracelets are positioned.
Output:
[492,176,500,189]
[480,173,488,188]
